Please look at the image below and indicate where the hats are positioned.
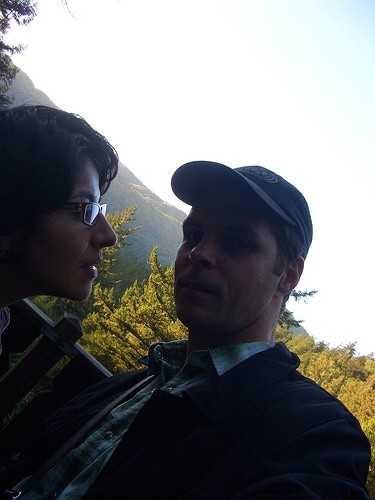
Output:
[170,160,314,263]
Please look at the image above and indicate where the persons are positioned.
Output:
[0,105,119,330]
[0,161,370,500]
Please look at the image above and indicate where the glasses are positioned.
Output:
[56,201,108,228]
